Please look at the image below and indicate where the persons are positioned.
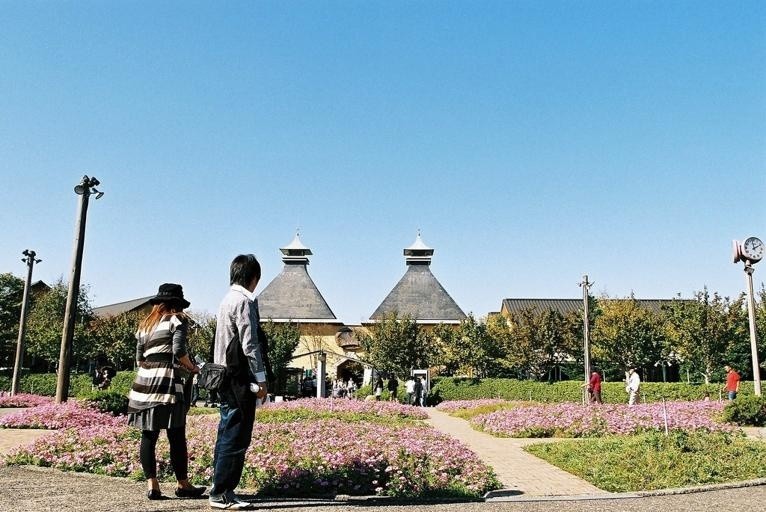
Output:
[588,366,604,404]
[622,364,644,406]
[297,373,357,400]
[125,282,208,499]
[373,371,429,409]
[90,367,102,390]
[208,254,270,509]
[99,368,113,390]
[191,371,200,407]
[720,363,742,401]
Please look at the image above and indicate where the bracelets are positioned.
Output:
[187,365,195,370]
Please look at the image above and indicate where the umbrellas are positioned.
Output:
[102,366,115,379]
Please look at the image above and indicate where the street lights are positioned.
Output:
[654,360,673,382]
[52,173,104,404]
[75,302,94,374]
[0,368,29,379]
[574,274,597,404]
[6,249,43,396]
[311,349,333,399]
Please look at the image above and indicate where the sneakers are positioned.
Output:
[210,488,253,510]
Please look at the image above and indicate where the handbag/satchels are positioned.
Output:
[198,363,227,392]
[172,349,195,374]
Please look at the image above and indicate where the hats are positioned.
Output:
[149,284,190,308]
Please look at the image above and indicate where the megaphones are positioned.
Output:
[74,182,86,195]
[91,187,104,200]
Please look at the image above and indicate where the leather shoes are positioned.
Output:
[175,484,206,497]
[148,489,161,500]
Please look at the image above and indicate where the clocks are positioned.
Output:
[741,236,766,264]
